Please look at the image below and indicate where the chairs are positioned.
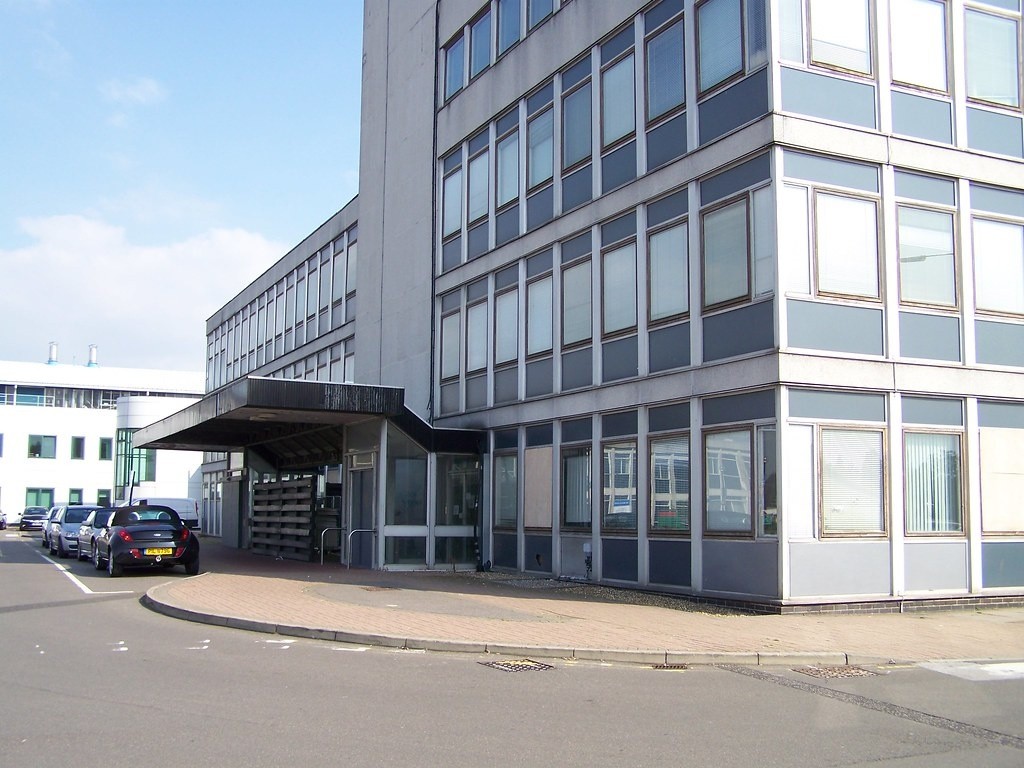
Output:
[128,512,141,521]
[28,510,109,526]
[157,511,170,519]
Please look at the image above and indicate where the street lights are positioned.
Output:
[720,467,725,511]
[764,456,767,510]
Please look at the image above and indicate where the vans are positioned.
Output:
[117,498,199,529]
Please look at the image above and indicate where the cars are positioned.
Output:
[18,506,49,530]
[47,505,106,558]
[42,506,63,548]
[93,505,199,577]
[0,510,7,530]
[502,470,514,478]
[604,512,637,528]
[77,507,116,564]
